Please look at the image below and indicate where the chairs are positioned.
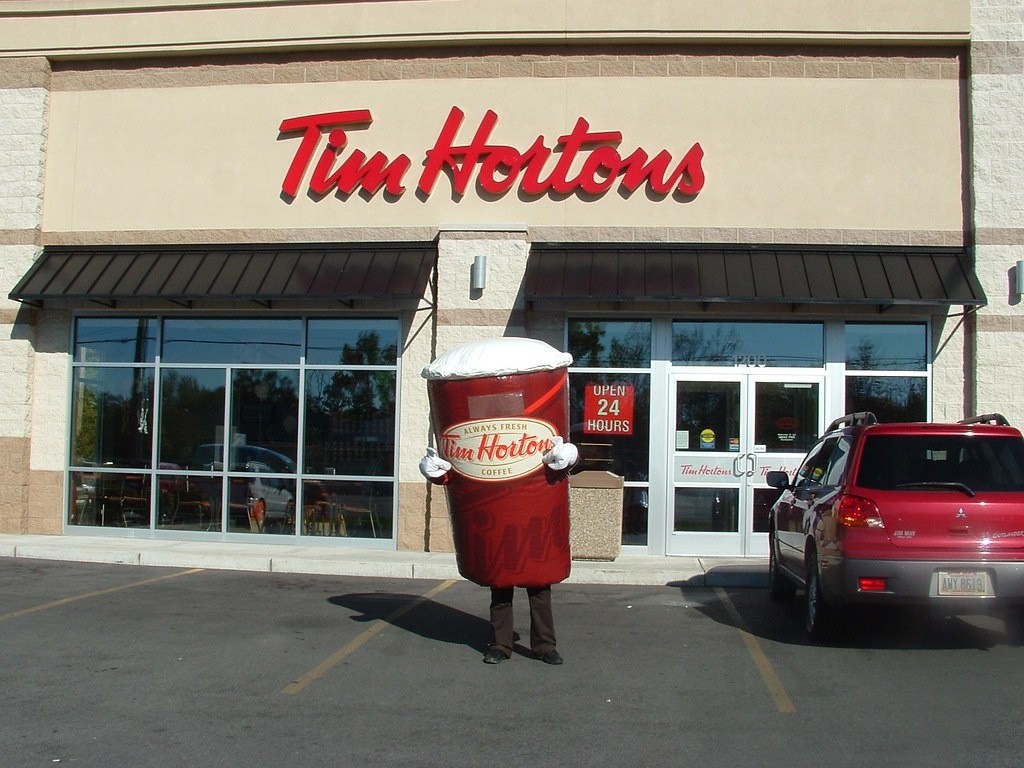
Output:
[73,461,384,537]
[955,443,993,492]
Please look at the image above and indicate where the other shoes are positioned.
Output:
[535,650,563,665]
[484,649,507,664]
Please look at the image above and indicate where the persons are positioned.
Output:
[420,336,585,665]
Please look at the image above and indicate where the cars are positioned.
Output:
[76,459,186,524]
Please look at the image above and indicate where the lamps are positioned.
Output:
[472,256,486,290]
[1015,261,1024,294]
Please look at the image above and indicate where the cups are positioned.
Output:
[421,351,574,587]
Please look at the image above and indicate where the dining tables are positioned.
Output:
[78,472,145,524]
[303,479,363,536]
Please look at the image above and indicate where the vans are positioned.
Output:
[184,443,296,526]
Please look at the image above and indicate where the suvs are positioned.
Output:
[765,412,1024,645]
